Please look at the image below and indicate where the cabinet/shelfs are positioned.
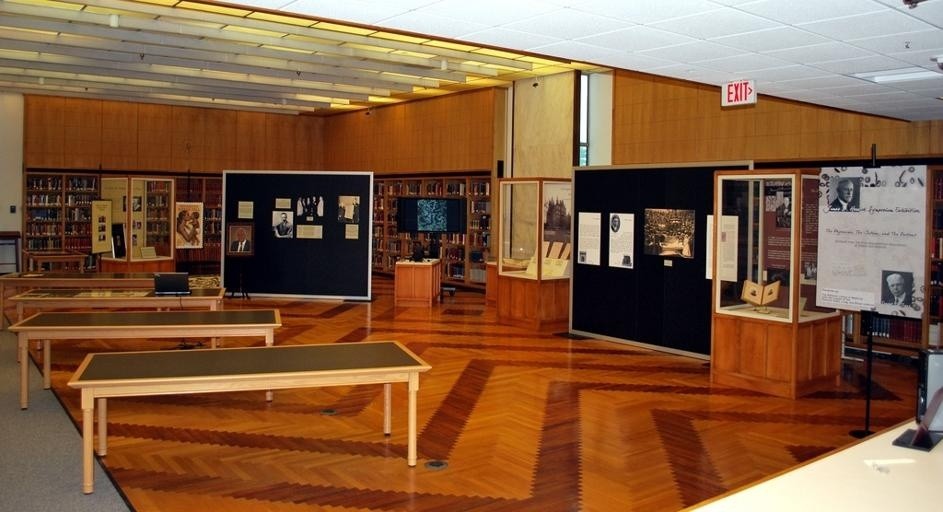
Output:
[23,170,99,277]
[374,173,491,292]
[99,173,222,278]
[852,166,943,359]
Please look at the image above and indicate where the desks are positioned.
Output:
[66,338,432,496]
[0,271,154,330]
[10,308,283,410]
[9,286,225,363]
[393,257,443,305]
[0,231,20,274]
[22,249,88,272]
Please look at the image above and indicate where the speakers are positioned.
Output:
[497,160,504,179]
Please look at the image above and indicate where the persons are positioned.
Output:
[829,179,856,211]
[231,229,250,253]
[881,272,912,304]
[190,212,201,246]
[133,199,139,211]
[178,211,195,245]
[272,213,293,238]
[612,217,619,231]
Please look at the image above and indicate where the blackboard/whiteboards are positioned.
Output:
[221,170,373,300]
[569,161,751,362]
[812,162,927,320]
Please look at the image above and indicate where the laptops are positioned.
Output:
[154,271,191,295]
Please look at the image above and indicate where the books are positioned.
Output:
[27,175,99,272]
[147,182,169,243]
[373,182,493,282]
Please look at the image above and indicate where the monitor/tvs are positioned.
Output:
[915,346,943,433]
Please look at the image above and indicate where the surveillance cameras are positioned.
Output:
[365,108,371,115]
[533,81,538,87]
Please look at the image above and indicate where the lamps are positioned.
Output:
[39,77,44,83]
[440,59,447,72]
[109,15,119,28]
